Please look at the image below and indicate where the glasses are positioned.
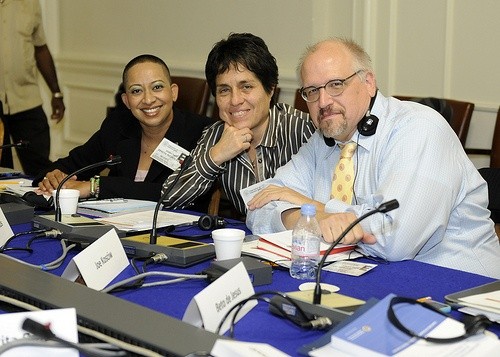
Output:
[300,69,362,104]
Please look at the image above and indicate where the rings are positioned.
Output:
[43,177,48,181]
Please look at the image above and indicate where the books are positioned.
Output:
[76,200,162,217]
[331,294,500,357]
[257,237,358,259]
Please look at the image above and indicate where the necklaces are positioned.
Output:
[251,155,256,169]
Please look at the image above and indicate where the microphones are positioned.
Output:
[0,139,30,151]
[267,199,399,331]
[32,156,123,242]
[118,154,216,269]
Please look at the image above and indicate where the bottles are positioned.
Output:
[290,204,321,279]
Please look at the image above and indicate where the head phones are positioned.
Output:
[322,89,379,148]
[0,191,54,209]
[388,296,491,344]
[163,214,225,242]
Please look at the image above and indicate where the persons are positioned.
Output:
[160,32,317,221]
[32,54,219,214]
[0,0,65,178]
[246,37,500,278]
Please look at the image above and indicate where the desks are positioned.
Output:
[0,196,500,357]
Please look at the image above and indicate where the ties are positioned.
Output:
[330,142,359,205]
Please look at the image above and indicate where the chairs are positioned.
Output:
[170,76,474,152]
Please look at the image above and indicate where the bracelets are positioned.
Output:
[90,176,99,197]
[53,92,63,97]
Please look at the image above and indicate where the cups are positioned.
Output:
[52,189,80,214]
[211,228,245,261]
